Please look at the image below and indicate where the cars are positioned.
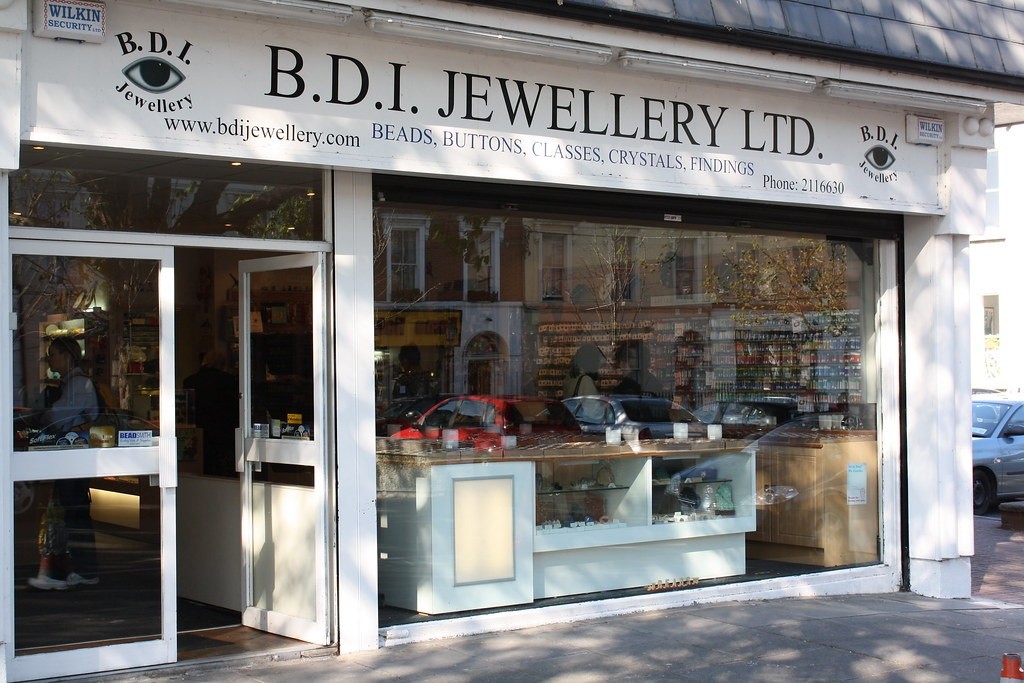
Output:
[378,391,801,442]
[972,380,1024,516]
[14,407,163,491]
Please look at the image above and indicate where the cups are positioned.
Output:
[488,423,501,433]
[501,435,517,449]
[442,429,458,450]
[388,424,401,437]
[707,424,722,439]
[819,415,832,429]
[520,423,532,435]
[673,422,688,439]
[831,415,843,429]
[605,426,622,445]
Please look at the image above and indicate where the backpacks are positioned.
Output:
[65,372,121,445]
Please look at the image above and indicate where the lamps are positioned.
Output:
[359,9,613,66]
[175,0,354,28]
[618,50,816,94]
[822,79,987,117]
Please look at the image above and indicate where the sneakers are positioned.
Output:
[67,572,100,586]
[28,576,68,590]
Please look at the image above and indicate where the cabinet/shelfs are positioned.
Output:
[36,316,109,392]
[690,429,877,568]
[90,429,203,547]
[377,438,760,615]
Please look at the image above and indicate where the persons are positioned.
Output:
[393,345,440,398]
[612,339,672,423]
[181,351,238,477]
[562,343,608,421]
[27,335,100,589]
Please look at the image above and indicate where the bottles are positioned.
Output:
[707,329,861,403]
